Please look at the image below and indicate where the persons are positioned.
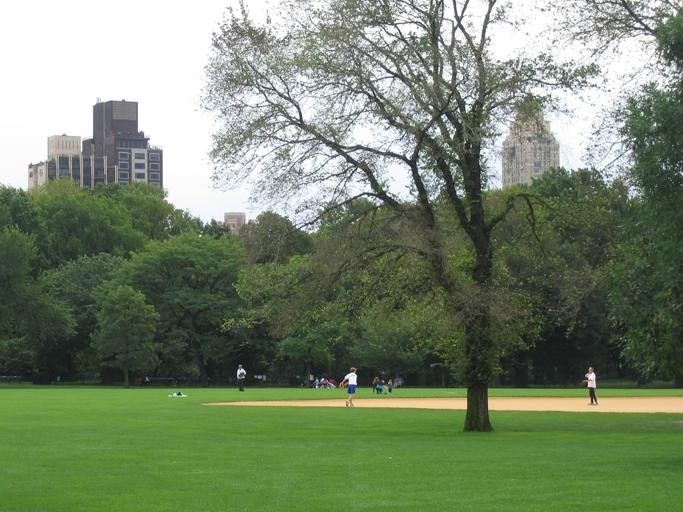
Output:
[236,364,246,391]
[310,366,404,407]
[582,366,598,405]
